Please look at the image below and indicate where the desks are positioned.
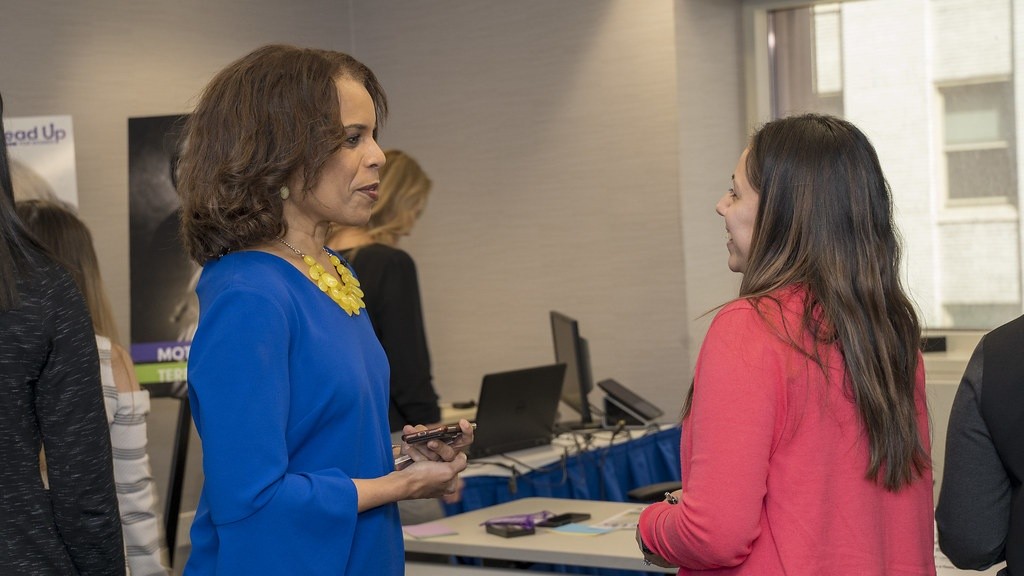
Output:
[437,422,683,518]
[401,497,1008,576]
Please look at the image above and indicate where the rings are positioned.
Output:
[640,557,652,567]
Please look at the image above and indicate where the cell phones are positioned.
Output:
[402,423,478,444]
[537,513,590,528]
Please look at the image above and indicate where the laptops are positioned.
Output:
[463,363,567,460]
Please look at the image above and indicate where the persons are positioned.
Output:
[183,44,474,575]
[0,95,164,576]
[636,112,936,576]
[934,313,1024,575]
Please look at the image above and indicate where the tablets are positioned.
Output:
[596,378,664,420]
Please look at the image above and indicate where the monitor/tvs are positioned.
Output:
[548,311,593,424]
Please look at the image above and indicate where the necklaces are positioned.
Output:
[275,235,367,318]
